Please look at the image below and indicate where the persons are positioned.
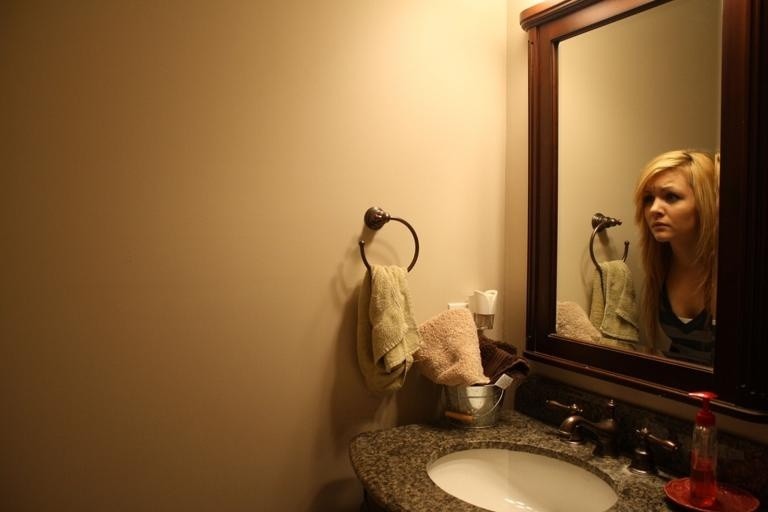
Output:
[632,148,720,371]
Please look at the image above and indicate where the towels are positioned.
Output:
[357,265,423,397]
[590,260,640,350]
[418,309,491,386]
[556,302,602,346]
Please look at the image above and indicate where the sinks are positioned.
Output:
[427,441,620,511]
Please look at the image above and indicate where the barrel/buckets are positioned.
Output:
[443,382,507,428]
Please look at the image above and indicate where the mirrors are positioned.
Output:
[520,0,768,424]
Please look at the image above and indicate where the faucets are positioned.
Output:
[558,399,621,459]
